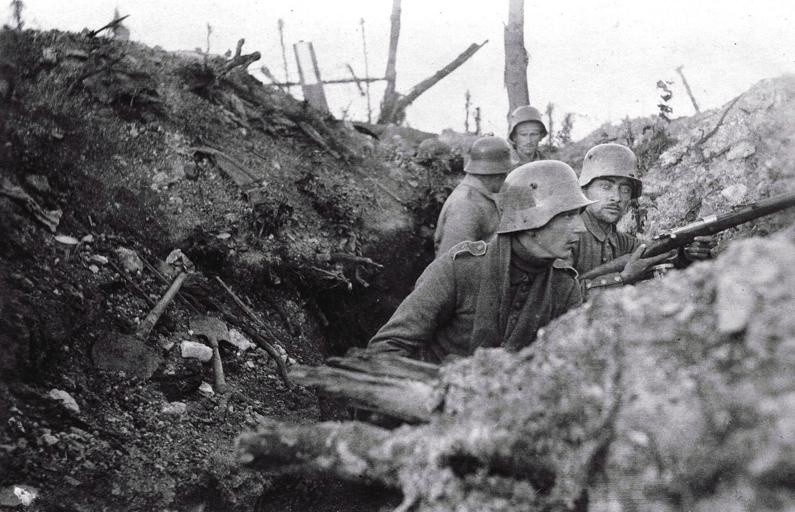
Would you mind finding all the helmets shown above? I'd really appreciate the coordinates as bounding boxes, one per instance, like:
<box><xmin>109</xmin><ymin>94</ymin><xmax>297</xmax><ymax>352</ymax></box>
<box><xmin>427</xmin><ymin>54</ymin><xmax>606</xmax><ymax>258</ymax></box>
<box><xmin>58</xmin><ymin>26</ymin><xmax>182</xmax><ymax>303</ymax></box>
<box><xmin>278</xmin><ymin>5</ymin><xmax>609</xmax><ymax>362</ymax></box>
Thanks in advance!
<box><xmin>507</xmin><ymin>107</ymin><xmax>548</xmax><ymax>140</ymax></box>
<box><xmin>497</xmin><ymin>159</ymin><xmax>599</xmax><ymax>233</ymax></box>
<box><xmin>416</xmin><ymin>138</ymin><xmax>451</xmax><ymax>164</ymax></box>
<box><xmin>578</xmin><ymin>143</ymin><xmax>643</xmax><ymax>198</ymax></box>
<box><xmin>464</xmin><ymin>136</ymin><xmax>513</xmax><ymax>176</ymax></box>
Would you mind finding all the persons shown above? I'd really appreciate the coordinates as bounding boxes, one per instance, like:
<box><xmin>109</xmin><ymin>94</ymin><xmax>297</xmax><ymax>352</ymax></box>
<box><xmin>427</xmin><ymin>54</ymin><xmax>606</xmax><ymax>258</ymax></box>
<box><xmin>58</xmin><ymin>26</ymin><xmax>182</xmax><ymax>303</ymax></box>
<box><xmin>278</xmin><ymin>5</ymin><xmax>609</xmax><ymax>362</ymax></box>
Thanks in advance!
<box><xmin>433</xmin><ymin>136</ymin><xmax>519</xmax><ymax>259</ymax></box>
<box><xmin>364</xmin><ymin>158</ymin><xmax>601</xmax><ymax>369</ymax></box>
<box><xmin>507</xmin><ymin>104</ymin><xmax>552</xmax><ymax>171</ymax></box>
<box><xmin>564</xmin><ymin>143</ymin><xmax>719</xmax><ymax>301</ymax></box>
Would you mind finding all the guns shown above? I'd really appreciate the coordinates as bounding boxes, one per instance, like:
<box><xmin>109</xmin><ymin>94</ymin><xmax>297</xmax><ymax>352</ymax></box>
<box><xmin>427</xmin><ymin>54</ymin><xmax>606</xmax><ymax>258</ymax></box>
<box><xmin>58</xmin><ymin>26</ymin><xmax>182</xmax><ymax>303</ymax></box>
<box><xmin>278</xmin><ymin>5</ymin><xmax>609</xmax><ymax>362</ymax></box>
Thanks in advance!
<box><xmin>577</xmin><ymin>191</ymin><xmax>795</xmax><ymax>280</ymax></box>
